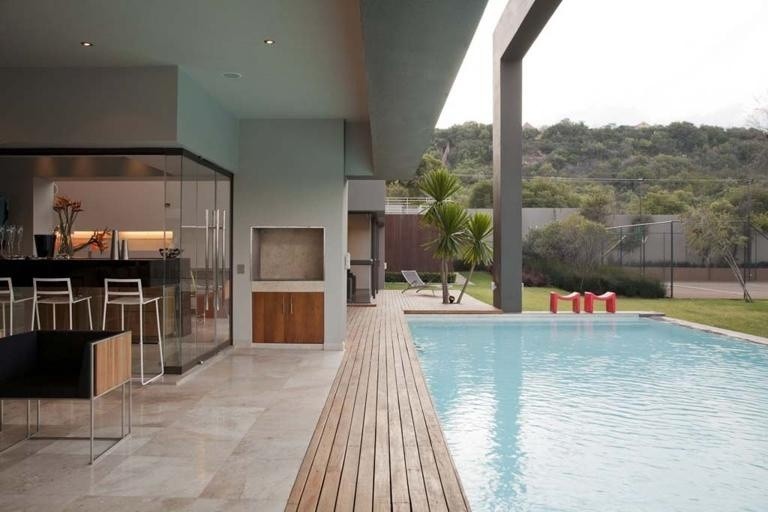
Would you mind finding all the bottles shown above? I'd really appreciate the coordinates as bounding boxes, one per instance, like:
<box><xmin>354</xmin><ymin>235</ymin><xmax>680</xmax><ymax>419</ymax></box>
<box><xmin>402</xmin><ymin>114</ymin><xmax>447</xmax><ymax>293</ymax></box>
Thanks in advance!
<box><xmin>110</xmin><ymin>230</ymin><xmax>120</xmax><ymax>260</ymax></box>
<box><xmin>120</xmin><ymin>239</ymin><xmax>128</xmax><ymax>260</ymax></box>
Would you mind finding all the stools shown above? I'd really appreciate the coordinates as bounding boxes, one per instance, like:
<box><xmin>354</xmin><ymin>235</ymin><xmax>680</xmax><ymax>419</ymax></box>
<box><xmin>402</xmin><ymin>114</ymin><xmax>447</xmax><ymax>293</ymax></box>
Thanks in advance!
<box><xmin>551</xmin><ymin>291</ymin><xmax>617</xmax><ymax>315</ymax></box>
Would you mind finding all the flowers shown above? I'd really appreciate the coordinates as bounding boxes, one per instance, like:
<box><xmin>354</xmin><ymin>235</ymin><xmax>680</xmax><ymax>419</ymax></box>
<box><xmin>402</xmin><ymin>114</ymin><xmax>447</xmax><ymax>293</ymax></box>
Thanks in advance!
<box><xmin>52</xmin><ymin>196</ymin><xmax>112</xmax><ymax>256</ymax></box>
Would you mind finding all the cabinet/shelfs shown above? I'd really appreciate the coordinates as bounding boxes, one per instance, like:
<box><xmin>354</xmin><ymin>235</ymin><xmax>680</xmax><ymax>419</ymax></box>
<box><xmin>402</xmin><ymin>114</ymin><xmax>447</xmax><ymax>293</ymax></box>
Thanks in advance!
<box><xmin>251</xmin><ymin>292</ymin><xmax>323</xmax><ymax>345</ymax></box>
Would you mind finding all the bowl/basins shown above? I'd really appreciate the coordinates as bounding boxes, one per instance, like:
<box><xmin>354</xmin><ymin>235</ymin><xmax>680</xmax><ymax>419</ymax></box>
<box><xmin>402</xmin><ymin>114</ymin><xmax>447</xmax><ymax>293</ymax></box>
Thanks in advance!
<box><xmin>158</xmin><ymin>248</ymin><xmax>184</xmax><ymax>258</ymax></box>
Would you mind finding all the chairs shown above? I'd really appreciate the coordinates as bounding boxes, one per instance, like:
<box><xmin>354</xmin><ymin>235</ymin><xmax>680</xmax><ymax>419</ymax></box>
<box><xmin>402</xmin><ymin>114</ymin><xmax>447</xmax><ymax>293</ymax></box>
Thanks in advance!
<box><xmin>1</xmin><ymin>329</ymin><xmax>131</xmax><ymax>470</ymax></box>
<box><xmin>401</xmin><ymin>270</ymin><xmax>439</xmax><ymax>298</ymax></box>
<box><xmin>0</xmin><ymin>276</ymin><xmax>167</xmax><ymax>388</ymax></box>
